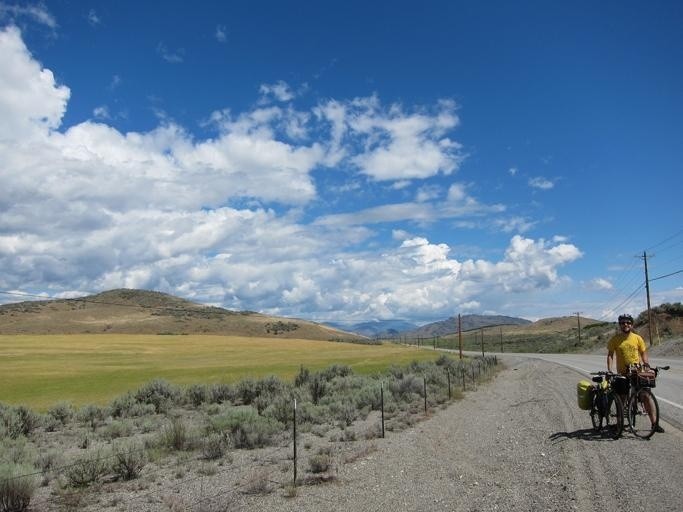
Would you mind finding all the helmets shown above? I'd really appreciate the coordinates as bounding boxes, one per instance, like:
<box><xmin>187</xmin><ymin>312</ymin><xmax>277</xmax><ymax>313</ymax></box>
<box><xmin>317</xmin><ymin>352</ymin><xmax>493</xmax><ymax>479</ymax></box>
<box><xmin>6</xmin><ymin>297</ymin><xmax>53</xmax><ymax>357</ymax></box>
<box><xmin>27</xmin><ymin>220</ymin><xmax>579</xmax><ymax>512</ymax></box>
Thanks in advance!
<box><xmin>618</xmin><ymin>314</ymin><xmax>634</xmax><ymax>323</ymax></box>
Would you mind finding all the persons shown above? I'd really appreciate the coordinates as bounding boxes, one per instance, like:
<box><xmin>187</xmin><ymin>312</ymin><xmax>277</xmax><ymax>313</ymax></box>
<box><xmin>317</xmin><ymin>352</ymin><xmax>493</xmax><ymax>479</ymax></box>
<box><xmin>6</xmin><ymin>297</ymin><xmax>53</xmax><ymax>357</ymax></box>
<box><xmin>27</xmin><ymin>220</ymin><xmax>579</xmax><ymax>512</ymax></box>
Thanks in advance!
<box><xmin>606</xmin><ymin>314</ymin><xmax>665</xmax><ymax>433</ymax></box>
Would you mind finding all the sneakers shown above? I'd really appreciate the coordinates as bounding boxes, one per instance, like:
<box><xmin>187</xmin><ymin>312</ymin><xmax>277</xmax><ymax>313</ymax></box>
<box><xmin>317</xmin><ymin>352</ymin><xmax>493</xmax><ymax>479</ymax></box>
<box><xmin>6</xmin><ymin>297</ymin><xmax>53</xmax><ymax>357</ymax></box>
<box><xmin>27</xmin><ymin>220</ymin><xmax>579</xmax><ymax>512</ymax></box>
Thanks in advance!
<box><xmin>651</xmin><ymin>424</ymin><xmax>664</xmax><ymax>433</ymax></box>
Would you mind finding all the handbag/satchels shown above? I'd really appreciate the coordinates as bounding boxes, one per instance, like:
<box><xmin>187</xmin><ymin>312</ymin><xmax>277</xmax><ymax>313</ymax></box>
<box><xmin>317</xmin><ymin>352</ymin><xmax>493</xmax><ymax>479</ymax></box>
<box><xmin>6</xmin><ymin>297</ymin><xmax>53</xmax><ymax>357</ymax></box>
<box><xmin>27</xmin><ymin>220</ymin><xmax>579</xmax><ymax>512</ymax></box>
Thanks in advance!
<box><xmin>577</xmin><ymin>380</ymin><xmax>594</xmax><ymax>410</ymax></box>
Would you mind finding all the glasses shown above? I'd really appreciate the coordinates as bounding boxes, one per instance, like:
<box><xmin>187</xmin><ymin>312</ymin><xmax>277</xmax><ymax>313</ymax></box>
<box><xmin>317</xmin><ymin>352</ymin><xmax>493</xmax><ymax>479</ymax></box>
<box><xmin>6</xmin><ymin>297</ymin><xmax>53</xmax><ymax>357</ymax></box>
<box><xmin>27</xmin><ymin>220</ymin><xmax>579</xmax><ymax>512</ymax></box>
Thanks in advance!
<box><xmin>621</xmin><ymin>321</ymin><xmax>632</xmax><ymax>325</ymax></box>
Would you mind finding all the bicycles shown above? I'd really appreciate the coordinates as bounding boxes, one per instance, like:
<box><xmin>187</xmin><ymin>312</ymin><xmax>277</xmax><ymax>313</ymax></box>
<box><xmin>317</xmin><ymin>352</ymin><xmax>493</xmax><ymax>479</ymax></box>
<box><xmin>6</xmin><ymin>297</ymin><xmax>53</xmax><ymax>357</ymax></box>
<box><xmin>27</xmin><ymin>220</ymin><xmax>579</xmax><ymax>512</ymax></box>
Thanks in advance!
<box><xmin>588</xmin><ymin>372</ymin><xmax>625</xmax><ymax>440</ymax></box>
<box><xmin>621</xmin><ymin>362</ymin><xmax>670</xmax><ymax>439</ymax></box>
